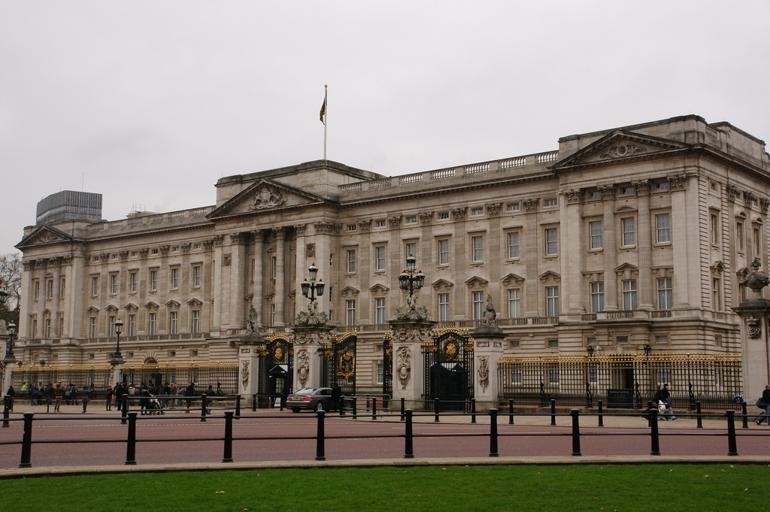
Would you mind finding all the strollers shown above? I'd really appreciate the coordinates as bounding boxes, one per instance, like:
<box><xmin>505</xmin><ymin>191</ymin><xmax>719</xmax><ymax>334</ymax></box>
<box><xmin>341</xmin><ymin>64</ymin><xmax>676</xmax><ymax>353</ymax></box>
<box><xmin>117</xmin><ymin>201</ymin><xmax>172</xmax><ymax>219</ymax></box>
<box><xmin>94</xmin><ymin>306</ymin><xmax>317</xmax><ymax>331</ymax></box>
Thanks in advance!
<box><xmin>148</xmin><ymin>393</ymin><xmax>164</xmax><ymax>416</ymax></box>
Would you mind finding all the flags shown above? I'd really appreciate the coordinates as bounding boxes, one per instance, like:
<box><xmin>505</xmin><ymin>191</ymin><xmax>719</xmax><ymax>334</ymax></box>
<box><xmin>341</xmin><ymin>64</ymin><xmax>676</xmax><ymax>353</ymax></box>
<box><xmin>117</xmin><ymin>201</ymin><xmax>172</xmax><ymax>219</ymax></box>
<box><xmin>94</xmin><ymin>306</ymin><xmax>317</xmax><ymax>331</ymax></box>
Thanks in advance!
<box><xmin>318</xmin><ymin>85</ymin><xmax>329</xmax><ymax>127</ymax></box>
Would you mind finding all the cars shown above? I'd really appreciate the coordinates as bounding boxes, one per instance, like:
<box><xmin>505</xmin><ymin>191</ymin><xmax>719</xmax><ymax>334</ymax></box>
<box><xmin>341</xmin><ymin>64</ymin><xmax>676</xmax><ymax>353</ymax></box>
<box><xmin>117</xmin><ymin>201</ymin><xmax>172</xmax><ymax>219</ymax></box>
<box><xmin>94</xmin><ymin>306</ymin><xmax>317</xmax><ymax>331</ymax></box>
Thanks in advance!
<box><xmin>285</xmin><ymin>387</ymin><xmax>353</xmax><ymax>412</ymax></box>
<box><xmin>65</xmin><ymin>385</ymin><xmax>92</xmax><ymax>399</ymax></box>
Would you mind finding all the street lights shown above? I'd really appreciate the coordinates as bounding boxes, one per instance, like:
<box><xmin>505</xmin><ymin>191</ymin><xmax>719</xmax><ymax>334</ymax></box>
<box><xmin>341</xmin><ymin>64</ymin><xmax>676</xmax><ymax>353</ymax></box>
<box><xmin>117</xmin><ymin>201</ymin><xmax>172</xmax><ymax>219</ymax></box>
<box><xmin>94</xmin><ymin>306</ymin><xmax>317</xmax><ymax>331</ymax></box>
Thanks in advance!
<box><xmin>300</xmin><ymin>262</ymin><xmax>324</xmax><ymax>315</ymax></box>
<box><xmin>113</xmin><ymin>317</ymin><xmax>124</xmax><ymax>351</ymax></box>
<box><xmin>587</xmin><ymin>343</ymin><xmax>594</xmax><ymax>357</ymax></box>
<box><xmin>5</xmin><ymin>320</ymin><xmax>16</xmax><ymax>354</ymax></box>
<box><xmin>399</xmin><ymin>252</ymin><xmax>426</xmax><ymax>309</ymax></box>
<box><xmin>643</xmin><ymin>342</ymin><xmax>651</xmax><ymax>355</ymax></box>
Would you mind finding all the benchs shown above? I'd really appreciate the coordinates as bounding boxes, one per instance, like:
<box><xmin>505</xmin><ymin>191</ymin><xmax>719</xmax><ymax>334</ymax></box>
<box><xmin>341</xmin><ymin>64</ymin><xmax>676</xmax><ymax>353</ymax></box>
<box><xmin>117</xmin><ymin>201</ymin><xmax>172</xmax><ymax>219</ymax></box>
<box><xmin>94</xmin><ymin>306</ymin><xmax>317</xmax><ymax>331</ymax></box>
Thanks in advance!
<box><xmin>11</xmin><ymin>398</ymin><xmax>107</xmax><ymax>406</ymax></box>
<box><xmin>129</xmin><ymin>399</ymin><xmax>235</xmax><ymax>408</ymax></box>
<box><xmin>500</xmin><ymin>403</ymin><xmax>731</xmax><ymax>419</ymax></box>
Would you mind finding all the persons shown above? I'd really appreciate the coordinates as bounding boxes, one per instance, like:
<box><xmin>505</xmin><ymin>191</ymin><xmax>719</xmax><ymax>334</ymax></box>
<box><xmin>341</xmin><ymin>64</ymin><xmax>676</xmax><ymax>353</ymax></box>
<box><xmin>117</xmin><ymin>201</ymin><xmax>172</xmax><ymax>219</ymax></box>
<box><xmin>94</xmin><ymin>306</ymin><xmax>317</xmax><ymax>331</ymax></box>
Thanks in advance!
<box><xmin>657</xmin><ymin>383</ymin><xmax>678</xmax><ymax>421</ymax></box>
<box><xmin>330</xmin><ymin>382</ymin><xmax>342</xmax><ymax>413</ymax></box>
<box><xmin>755</xmin><ymin>384</ymin><xmax>769</xmax><ymax>425</ymax></box>
<box><xmin>4</xmin><ymin>376</ymin><xmax>226</xmax><ymax>417</ymax></box>
